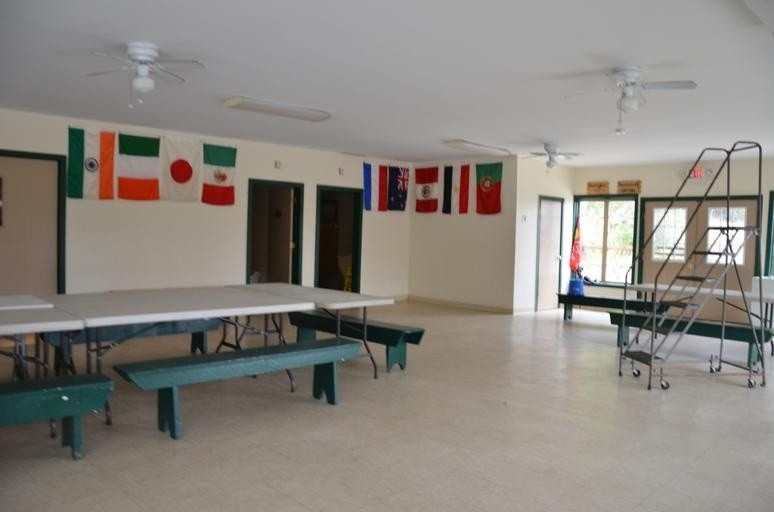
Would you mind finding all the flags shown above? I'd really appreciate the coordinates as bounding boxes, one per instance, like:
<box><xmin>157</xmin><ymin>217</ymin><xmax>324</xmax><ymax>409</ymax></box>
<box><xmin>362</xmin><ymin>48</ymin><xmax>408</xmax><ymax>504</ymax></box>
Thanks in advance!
<box><xmin>202</xmin><ymin>144</ymin><xmax>237</xmax><ymax>206</ymax></box>
<box><xmin>415</xmin><ymin>167</ymin><xmax>438</xmax><ymax>211</ymax></box>
<box><xmin>476</xmin><ymin>162</ymin><xmax>503</xmax><ymax>215</ymax></box>
<box><xmin>570</xmin><ymin>215</ymin><xmax>585</xmax><ymax>272</ymax></box>
<box><xmin>363</xmin><ymin>163</ymin><xmax>389</xmax><ymax>211</ymax></box>
<box><xmin>388</xmin><ymin>166</ymin><xmax>410</xmax><ymax>210</ymax></box>
<box><xmin>160</xmin><ymin>135</ymin><xmax>204</xmax><ymax>202</ymax></box>
<box><xmin>442</xmin><ymin>164</ymin><xmax>469</xmax><ymax>213</ymax></box>
<box><xmin>67</xmin><ymin>127</ymin><xmax>114</xmax><ymax>201</ymax></box>
<box><xmin>116</xmin><ymin>132</ymin><xmax>163</xmax><ymax>201</ymax></box>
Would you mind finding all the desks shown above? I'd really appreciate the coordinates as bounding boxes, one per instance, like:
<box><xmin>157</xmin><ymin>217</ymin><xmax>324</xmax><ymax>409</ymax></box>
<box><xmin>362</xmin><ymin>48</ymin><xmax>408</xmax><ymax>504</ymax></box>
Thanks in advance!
<box><xmin>0</xmin><ymin>284</ymin><xmax>315</xmax><ymax>442</ymax></box>
<box><xmin>227</xmin><ymin>281</ymin><xmax>394</xmax><ymax>380</ymax></box>
<box><xmin>630</xmin><ymin>284</ymin><xmax>773</xmax><ymax>363</ymax></box>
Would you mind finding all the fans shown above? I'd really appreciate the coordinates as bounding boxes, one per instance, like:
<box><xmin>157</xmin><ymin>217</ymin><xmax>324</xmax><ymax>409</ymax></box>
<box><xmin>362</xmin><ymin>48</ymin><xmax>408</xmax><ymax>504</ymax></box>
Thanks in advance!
<box><xmin>78</xmin><ymin>42</ymin><xmax>200</xmax><ymax>110</ymax></box>
<box><xmin>531</xmin><ymin>142</ymin><xmax>579</xmax><ymax>169</ymax></box>
<box><xmin>609</xmin><ymin>66</ymin><xmax>695</xmax><ymax>136</ymax></box>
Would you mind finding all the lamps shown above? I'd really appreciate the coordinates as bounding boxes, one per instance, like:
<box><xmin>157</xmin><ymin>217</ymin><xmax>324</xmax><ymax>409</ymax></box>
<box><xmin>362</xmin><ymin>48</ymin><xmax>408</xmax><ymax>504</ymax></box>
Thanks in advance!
<box><xmin>750</xmin><ymin>276</ymin><xmax>774</xmax><ymax>326</ymax></box>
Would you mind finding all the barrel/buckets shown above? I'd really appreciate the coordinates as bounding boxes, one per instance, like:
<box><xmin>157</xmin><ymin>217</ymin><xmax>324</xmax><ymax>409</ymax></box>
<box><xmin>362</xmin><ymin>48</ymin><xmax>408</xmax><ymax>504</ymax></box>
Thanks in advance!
<box><xmin>567</xmin><ymin>279</ymin><xmax>584</xmax><ymax>296</ymax></box>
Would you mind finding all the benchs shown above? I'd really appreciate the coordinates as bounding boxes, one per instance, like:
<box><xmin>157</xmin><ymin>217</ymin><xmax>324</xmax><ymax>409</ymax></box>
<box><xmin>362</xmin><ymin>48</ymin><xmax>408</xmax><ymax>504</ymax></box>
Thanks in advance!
<box><xmin>40</xmin><ymin>317</ymin><xmax>224</xmax><ymax>366</ymax></box>
<box><xmin>2</xmin><ymin>367</ymin><xmax>118</xmax><ymax>460</ymax></box>
<box><xmin>558</xmin><ymin>294</ymin><xmax>773</xmax><ymax>362</ymax></box>
<box><xmin>288</xmin><ymin>311</ymin><xmax>424</xmax><ymax>374</ymax></box>
<box><xmin>111</xmin><ymin>337</ymin><xmax>365</xmax><ymax>440</ymax></box>
